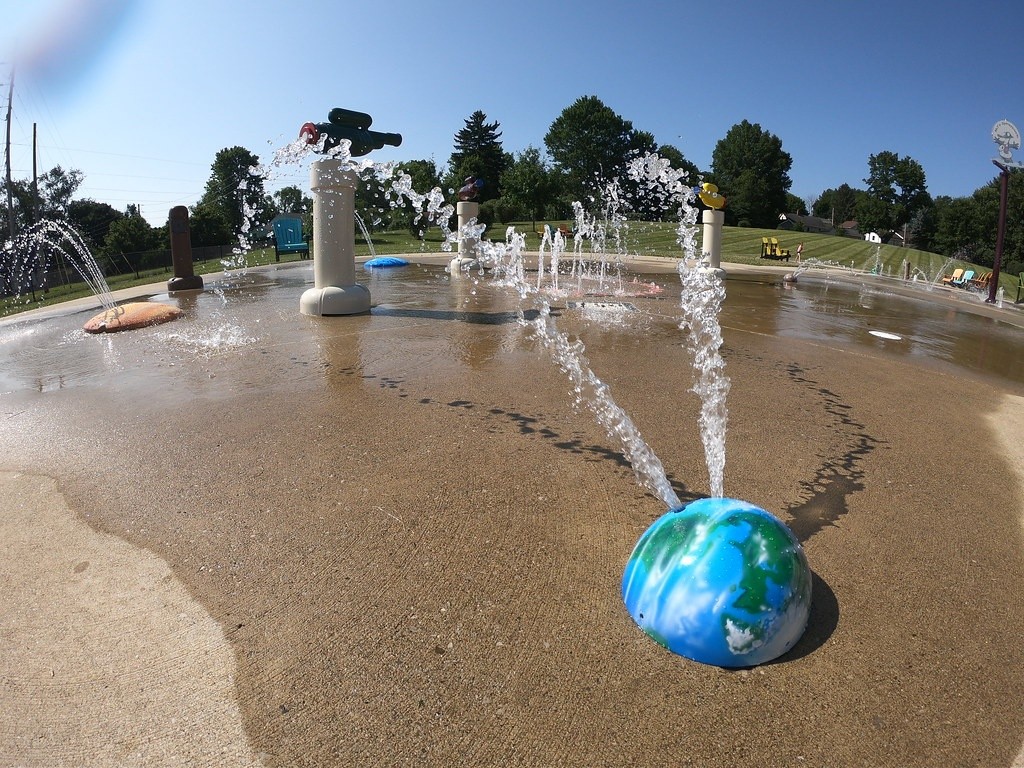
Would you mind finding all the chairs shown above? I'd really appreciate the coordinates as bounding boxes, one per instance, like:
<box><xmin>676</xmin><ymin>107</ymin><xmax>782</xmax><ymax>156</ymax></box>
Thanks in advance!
<box><xmin>760</xmin><ymin>236</ymin><xmax>791</xmax><ymax>262</ymax></box>
<box><xmin>536</xmin><ymin>223</ymin><xmax>575</xmax><ymax>239</ymax></box>
<box><xmin>271</xmin><ymin>215</ymin><xmax>311</xmax><ymax>261</ymax></box>
<box><xmin>1015</xmin><ymin>272</ymin><xmax>1024</xmax><ymax>303</ymax></box>
<box><xmin>943</xmin><ymin>269</ymin><xmax>992</xmax><ymax>292</ymax></box>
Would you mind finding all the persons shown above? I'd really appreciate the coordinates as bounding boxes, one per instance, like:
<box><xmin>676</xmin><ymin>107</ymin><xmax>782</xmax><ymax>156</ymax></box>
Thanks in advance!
<box><xmin>571</xmin><ymin>223</ymin><xmax>578</xmax><ymax>237</ymax></box>
<box><xmin>795</xmin><ymin>242</ymin><xmax>803</xmax><ymax>263</ymax></box>
